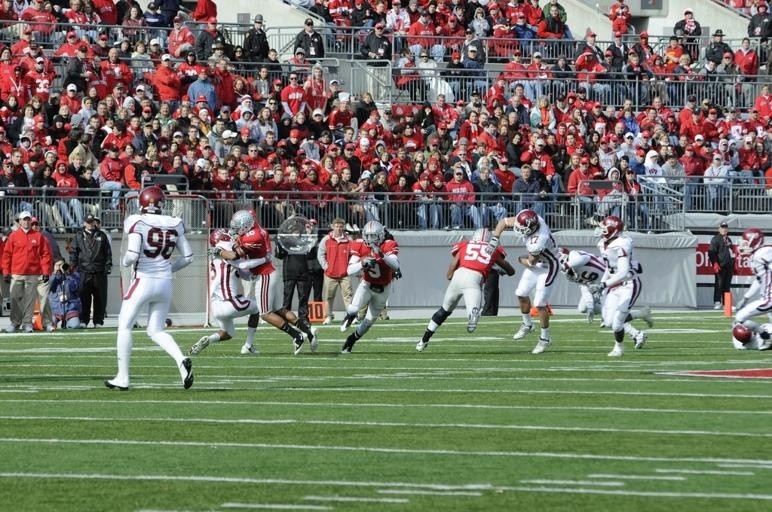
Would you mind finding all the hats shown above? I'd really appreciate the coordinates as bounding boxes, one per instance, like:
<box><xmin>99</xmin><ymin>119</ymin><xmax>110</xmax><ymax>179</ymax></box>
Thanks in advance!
<box><xmin>15</xmin><ymin>210</ymin><xmax>39</xmax><ymax>224</ymax></box>
<box><xmin>0</xmin><ymin>0</ymin><xmax>758</xmax><ymax>174</ymax></box>
<box><xmin>720</xmin><ymin>223</ymin><xmax>728</xmax><ymax>227</ymax></box>
<box><xmin>84</xmin><ymin>214</ymin><xmax>100</xmax><ymax>222</ymax></box>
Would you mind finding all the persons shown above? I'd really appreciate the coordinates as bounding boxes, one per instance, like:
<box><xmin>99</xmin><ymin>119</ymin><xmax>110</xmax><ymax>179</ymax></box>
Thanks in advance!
<box><xmin>186</xmin><ymin>226</ymin><xmax>274</xmax><ymax>358</ymax></box>
<box><xmin>414</xmin><ymin>226</ymin><xmax>515</xmax><ymax>351</ymax></box>
<box><xmin>484</xmin><ymin>207</ymin><xmax>561</xmax><ymax>355</ymax></box>
<box><xmin>726</xmin><ymin>321</ymin><xmax>771</xmax><ymax>350</ymax></box>
<box><xmin>209</xmin><ymin>207</ymin><xmax>318</xmax><ymax>355</ymax></box>
<box><xmin>597</xmin><ymin>217</ymin><xmax>648</xmax><ymax>358</ymax></box>
<box><xmin>103</xmin><ymin>186</ymin><xmax>196</xmax><ymax>392</ymax></box>
<box><xmin>0</xmin><ymin>1</ymin><xmax>771</xmax><ymax>334</ymax></box>
<box><xmin>338</xmin><ymin>219</ymin><xmax>402</xmax><ymax>356</ymax></box>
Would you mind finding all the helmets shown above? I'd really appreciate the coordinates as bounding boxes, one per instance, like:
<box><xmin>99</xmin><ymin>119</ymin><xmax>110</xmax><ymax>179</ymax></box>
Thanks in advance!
<box><xmin>473</xmin><ymin>228</ymin><xmax>491</xmax><ymax>241</ymax></box>
<box><xmin>593</xmin><ymin>216</ymin><xmax>623</xmax><ymax>242</ymax></box>
<box><xmin>733</xmin><ymin>323</ymin><xmax>752</xmax><ymax>343</ymax></box>
<box><xmin>513</xmin><ymin>209</ymin><xmax>538</xmax><ymax>237</ymax></box>
<box><xmin>362</xmin><ymin>221</ymin><xmax>382</xmax><ymax>247</ymax></box>
<box><xmin>741</xmin><ymin>228</ymin><xmax>763</xmax><ymax>253</ymax></box>
<box><xmin>209</xmin><ymin>210</ymin><xmax>256</xmax><ymax>247</ymax></box>
<box><xmin>138</xmin><ymin>186</ymin><xmax>165</xmax><ymax>214</ymax></box>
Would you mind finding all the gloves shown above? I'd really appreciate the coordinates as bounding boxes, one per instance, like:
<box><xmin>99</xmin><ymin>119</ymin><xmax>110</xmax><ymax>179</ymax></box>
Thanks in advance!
<box><xmin>485</xmin><ymin>237</ymin><xmax>499</xmax><ymax>255</ymax></box>
<box><xmin>713</xmin><ymin>263</ymin><xmax>721</xmax><ymax>273</ymax></box>
<box><xmin>589</xmin><ymin>283</ymin><xmax>604</xmax><ymax>298</ymax></box>
<box><xmin>362</xmin><ymin>244</ymin><xmax>382</xmax><ymax>271</ymax></box>
<box><xmin>736</xmin><ymin>297</ymin><xmax>746</xmax><ymax>310</ymax></box>
<box><xmin>586</xmin><ymin>309</ymin><xmax>595</xmax><ymax>324</ymax></box>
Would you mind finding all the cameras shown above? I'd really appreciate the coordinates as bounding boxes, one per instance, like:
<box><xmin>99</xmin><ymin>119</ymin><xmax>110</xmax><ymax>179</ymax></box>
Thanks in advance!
<box><xmin>59</xmin><ymin>263</ymin><xmax>69</xmax><ymax>274</ymax></box>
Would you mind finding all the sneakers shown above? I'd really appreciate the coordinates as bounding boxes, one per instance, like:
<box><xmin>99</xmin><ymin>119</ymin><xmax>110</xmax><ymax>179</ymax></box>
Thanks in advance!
<box><xmin>514</xmin><ymin>322</ymin><xmax>534</xmax><ymax>340</ymax></box>
<box><xmin>179</xmin><ymin>357</ymin><xmax>194</xmax><ymax>389</ymax></box>
<box><xmin>600</xmin><ymin>305</ymin><xmax>653</xmax><ymax>356</ymax></box>
<box><xmin>189</xmin><ymin>336</ymin><xmax>209</xmax><ymax>355</ymax></box>
<box><xmin>6</xmin><ymin>322</ymin><xmax>103</xmax><ymax>333</ymax></box>
<box><xmin>416</xmin><ymin>338</ymin><xmax>429</xmax><ymax>351</ymax></box>
<box><xmin>468</xmin><ymin>307</ymin><xmax>479</xmax><ymax>332</ymax></box>
<box><xmin>105</xmin><ymin>379</ymin><xmax>128</xmax><ymax>391</ymax></box>
<box><xmin>714</xmin><ymin>301</ymin><xmax>735</xmax><ymax>310</ymax></box>
<box><xmin>531</xmin><ymin>336</ymin><xmax>550</xmax><ymax>354</ymax></box>
<box><xmin>241</xmin><ymin>316</ymin><xmax>361</xmax><ymax>354</ymax></box>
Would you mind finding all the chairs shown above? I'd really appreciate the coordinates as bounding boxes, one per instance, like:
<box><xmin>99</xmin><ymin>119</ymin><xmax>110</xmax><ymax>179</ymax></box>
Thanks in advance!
<box><xmin>0</xmin><ymin>0</ymin><xmax>772</xmax><ymax>238</ymax></box>
